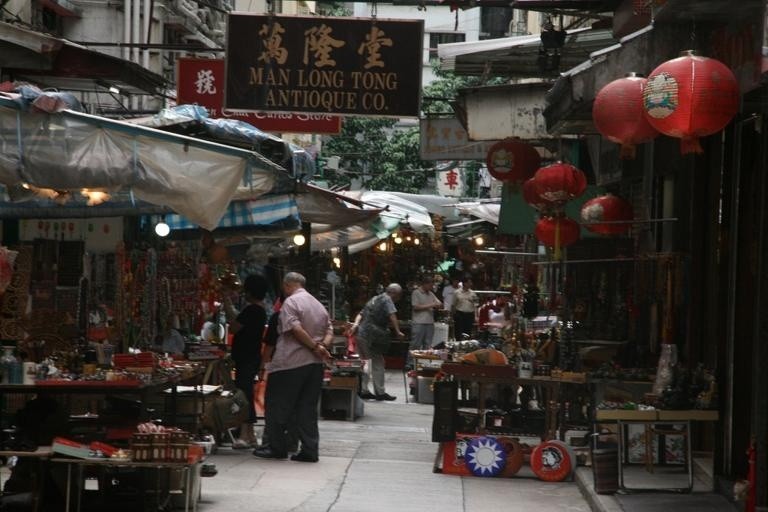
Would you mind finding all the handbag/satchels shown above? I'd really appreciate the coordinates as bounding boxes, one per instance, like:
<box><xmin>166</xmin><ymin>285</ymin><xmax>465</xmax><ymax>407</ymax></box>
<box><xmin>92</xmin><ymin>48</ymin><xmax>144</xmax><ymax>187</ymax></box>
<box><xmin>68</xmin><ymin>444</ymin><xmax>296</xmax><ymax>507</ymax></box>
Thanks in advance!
<box><xmin>367</xmin><ymin>324</ymin><xmax>392</xmax><ymax>353</ymax></box>
<box><xmin>202</xmin><ymin>389</ymin><xmax>251</xmax><ymax>434</ymax></box>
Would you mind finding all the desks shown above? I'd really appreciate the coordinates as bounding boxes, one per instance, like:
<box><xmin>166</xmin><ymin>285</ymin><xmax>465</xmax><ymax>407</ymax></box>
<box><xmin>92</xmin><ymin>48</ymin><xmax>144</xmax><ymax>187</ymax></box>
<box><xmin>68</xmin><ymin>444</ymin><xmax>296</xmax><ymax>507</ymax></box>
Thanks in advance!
<box><xmin>408</xmin><ymin>348</ymin><xmax>562</xmax><ymax>480</ymax></box>
<box><xmin>0</xmin><ymin>362</ymin><xmax>223</xmax><ymax>512</ymax></box>
<box><xmin>591</xmin><ymin>407</ymin><xmax>721</xmax><ymax>497</ymax></box>
<box><xmin>317</xmin><ymin>375</ymin><xmax>359</xmax><ymax>422</ymax></box>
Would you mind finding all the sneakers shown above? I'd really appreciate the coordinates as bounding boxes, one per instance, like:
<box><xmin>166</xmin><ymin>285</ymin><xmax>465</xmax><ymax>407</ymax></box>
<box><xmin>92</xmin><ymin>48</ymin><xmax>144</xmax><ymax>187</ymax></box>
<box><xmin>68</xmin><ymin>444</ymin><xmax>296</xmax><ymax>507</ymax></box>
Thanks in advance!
<box><xmin>360</xmin><ymin>391</ymin><xmax>376</xmax><ymax>400</ymax></box>
<box><xmin>233</xmin><ymin>435</ymin><xmax>256</xmax><ymax>449</ymax></box>
<box><xmin>290</xmin><ymin>452</ymin><xmax>319</xmax><ymax>462</ymax></box>
<box><xmin>252</xmin><ymin>444</ymin><xmax>289</xmax><ymax>459</ymax></box>
<box><xmin>376</xmin><ymin>393</ymin><xmax>397</xmax><ymax>401</ymax></box>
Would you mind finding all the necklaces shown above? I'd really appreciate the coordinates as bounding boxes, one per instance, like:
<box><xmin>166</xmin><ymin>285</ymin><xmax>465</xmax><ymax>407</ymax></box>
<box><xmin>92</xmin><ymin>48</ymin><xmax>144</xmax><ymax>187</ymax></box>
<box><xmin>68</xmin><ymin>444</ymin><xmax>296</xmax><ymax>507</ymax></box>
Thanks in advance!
<box><xmin>74</xmin><ymin>242</ymin><xmax>174</xmax><ymax>348</ymax></box>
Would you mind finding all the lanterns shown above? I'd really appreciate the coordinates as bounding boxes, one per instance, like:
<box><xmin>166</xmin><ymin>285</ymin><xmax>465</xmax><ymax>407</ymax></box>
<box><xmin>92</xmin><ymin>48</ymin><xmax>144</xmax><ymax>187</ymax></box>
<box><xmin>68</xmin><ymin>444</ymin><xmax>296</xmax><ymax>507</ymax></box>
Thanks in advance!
<box><xmin>487</xmin><ymin>135</ymin><xmax>538</xmax><ymax>189</ymax></box>
<box><xmin>640</xmin><ymin>48</ymin><xmax>738</xmax><ymax>154</ymax></box>
<box><xmin>593</xmin><ymin>72</ymin><xmax>655</xmax><ymax>157</ymax></box>
<box><xmin>523</xmin><ymin>158</ymin><xmax>634</xmax><ymax>263</ymax></box>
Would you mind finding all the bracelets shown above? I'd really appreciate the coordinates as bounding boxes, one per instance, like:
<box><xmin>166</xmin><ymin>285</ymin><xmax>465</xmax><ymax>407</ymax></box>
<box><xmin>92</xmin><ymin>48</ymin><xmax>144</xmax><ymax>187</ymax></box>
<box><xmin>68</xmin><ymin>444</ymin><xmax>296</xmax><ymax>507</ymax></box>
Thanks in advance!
<box><xmin>258</xmin><ymin>368</ymin><xmax>267</xmax><ymax>372</ymax></box>
<box><xmin>320</xmin><ymin>342</ymin><xmax>327</xmax><ymax>350</ymax></box>
<box><xmin>312</xmin><ymin>342</ymin><xmax>319</xmax><ymax>352</ymax></box>
<box><xmin>174</xmin><ymin>249</ymin><xmax>203</xmax><ymax>316</ymax></box>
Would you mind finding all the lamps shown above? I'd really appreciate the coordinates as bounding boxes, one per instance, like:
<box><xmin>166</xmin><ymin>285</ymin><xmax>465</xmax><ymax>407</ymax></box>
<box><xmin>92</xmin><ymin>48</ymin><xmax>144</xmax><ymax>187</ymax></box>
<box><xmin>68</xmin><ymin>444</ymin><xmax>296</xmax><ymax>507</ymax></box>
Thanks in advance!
<box><xmin>21</xmin><ymin>182</ymin><xmax>112</xmax><ymax>208</ymax></box>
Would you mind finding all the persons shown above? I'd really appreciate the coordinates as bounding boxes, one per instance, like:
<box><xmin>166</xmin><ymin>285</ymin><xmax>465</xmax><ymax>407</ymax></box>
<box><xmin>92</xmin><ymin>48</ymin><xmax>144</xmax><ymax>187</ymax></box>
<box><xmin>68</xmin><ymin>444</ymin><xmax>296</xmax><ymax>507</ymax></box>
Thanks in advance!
<box><xmin>256</xmin><ymin>298</ymin><xmax>296</xmax><ymax>459</ymax></box>
<box><xmin>254</xmin><ymin>271</ymin><xmax>333</xmax><ymax>462</ymax></box>
<box><xmin>346</xmin><ymin>283</ymin><xmax>405</xmax><ymax>400</ymax></box>
<box><xmin>403</xmin><ymin>275</ymin><xmax>441</xmax><ymax>371</ymax></box>
<box><xmin>442</xmin><ymin>277</ymin><xmax>459</xmax><ymax>339</ymax></box>
<box><xmin>453</xmin><ymin>436</ymin><xmax>473</xmax><ymax>466</ymax></box>
<box><xmin>224</xmin><ymin>275</ymin><xmax>266</xmax><ymax>449</ymax></box>
<box><xmin>538</xmin><ymin>446</ymin><xmax>562</xmax><ymax>472</ymax></box>
<box><xmin>479</xmin><ymin>288</ymin><xmax>512</xmax><ymax>337</ymax></box>
<box><xmin>450</xmin><ymin>278</ymin><xmax>478</xmax><ymax>341</ymax></box>
